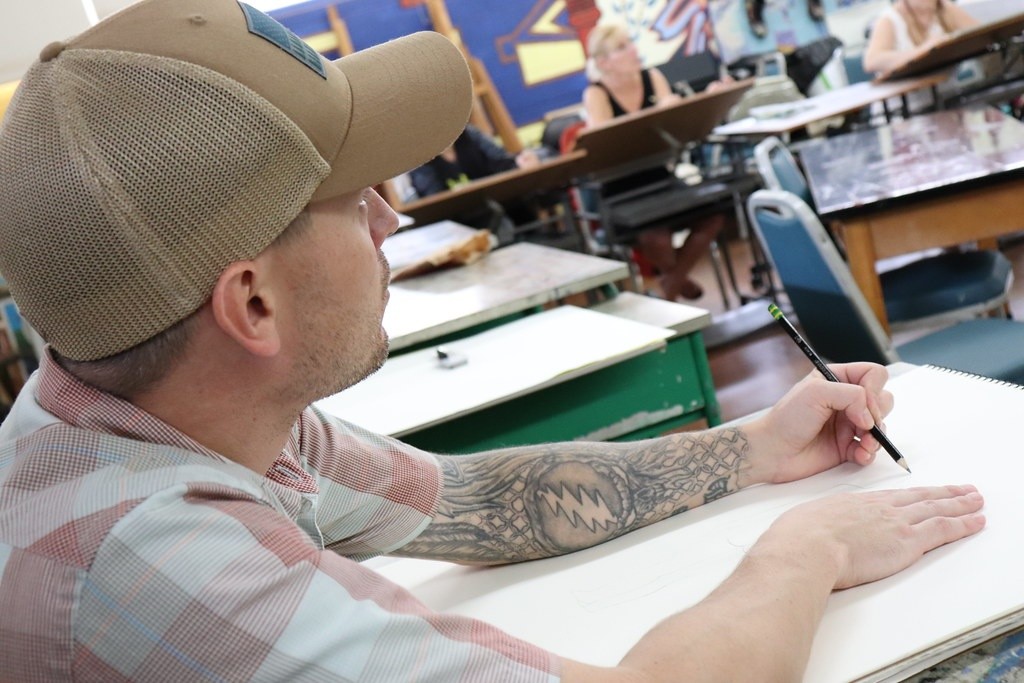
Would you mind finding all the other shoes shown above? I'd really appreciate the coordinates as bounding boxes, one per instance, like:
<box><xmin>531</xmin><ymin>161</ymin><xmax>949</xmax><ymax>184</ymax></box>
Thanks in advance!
<box><xmin>676</xmin><ymin>272</ymin><xmax>702</xmax><ymax>299</ymax></box>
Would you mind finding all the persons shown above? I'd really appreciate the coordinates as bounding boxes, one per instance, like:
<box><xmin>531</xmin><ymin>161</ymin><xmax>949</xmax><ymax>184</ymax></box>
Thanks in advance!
<box><xmin>863</xmin><ymin>0</ymin><xmax>985</xmax><ymax>128</ymax></box>
<box><xmin>582</xmin><ymin>22</ymin><xmax>727</xmax><ymax>302</ymax></box>
<box><xmin>410</xmin><ymin>125</ymin><xmax>541</xmax><ymax>244</ymax></box>
<box><xmin>0</xmin><ymin>0</ymin><xmax>986</xmax><ymax>683</ymax></box>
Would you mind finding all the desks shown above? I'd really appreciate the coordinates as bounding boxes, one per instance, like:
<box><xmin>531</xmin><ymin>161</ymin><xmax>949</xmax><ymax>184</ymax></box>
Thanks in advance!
<box><xmin>313</xmin><ymin>0</ymin><xmax>1024</xmax><ymax>683</ymax></box>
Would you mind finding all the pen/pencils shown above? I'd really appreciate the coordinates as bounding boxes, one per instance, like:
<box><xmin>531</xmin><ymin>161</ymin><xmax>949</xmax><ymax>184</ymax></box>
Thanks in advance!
<box><xmin>767</xmin><ymin>303</ymin><xmax>912</xmax><ymax>474</ymax></box>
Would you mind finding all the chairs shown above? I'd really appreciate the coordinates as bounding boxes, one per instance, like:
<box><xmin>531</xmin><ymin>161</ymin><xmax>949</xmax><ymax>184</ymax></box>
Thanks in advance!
<box><xmin>750</xmin><ymin>135</ymin><xmax>1024</xmax><ymax>332</ymax></box>
<box><xmin>563</xmin><ymin>121</ymin><xmax>742</xmax><ymax>314</ymax></box>
<box><xmin>746</xmin><ymin>189</ymin><xmax>1024</xmax><ymax>386</ymax></box>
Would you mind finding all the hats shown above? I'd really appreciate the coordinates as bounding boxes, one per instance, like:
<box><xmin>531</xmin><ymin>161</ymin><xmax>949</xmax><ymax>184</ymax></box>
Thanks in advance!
<box><xmin>0</xmin><ymin>0</ymin><xmax>469</xmax><ymax>362</ymax></box>
